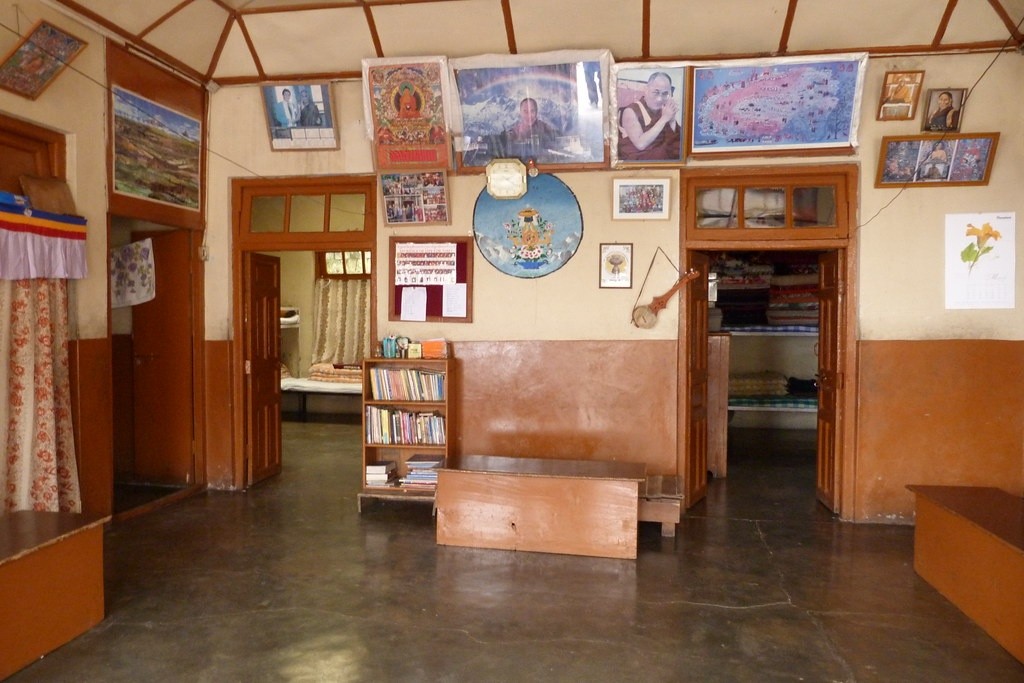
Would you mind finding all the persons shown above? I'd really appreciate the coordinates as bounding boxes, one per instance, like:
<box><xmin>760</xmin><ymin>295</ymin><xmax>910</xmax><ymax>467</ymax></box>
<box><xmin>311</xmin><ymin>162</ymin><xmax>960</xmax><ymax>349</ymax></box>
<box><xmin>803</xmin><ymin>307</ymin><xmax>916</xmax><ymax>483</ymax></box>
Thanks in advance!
<box><xmin>502</xmin><ymin>98</ymin><xmax>568</xmax><ymax>145</ymax></box>
<box><xmin>619</xmin><ymin>73</ymin><xmax>683</xmax><ymax>160</ymax></box>
<box><xmin>384</xmin><ymin>175</ymin><xmax>448</xmax><ymax>223</ymax></box>
<box><xmin>277</xmin><ymin>89</ymin><xmax>303</xmax><ymax>128</ymax></box>
<box><xmin>294</xmin><ymin>90</ymin><xmax>323</xmax><ymax>129</ymax></box>
<box><xmin>620</xmin><ymin>186</ymin><xmax>664</xmax><ymax>214</ymax></box>
<box><xmin>920</xmin><ymin>142</ymin><xmax>950</xmax><ymax>181</ymax></box>
<box><xmin>891</xmin><ymin>80</ymin><xmax>911</xmax><ymax>103</ymax></box>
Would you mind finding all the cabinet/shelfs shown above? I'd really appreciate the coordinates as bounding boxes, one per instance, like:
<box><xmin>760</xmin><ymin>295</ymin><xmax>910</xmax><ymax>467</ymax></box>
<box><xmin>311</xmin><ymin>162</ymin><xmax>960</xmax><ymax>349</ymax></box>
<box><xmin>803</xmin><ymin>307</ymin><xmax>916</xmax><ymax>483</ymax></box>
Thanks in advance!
<box><xmin>430</xmin><ymin>453</ymin><xmax>648</xmax><ymax>560</ymax></box>
<box><xmin>903</xmin><ymin>483</ymin><xmax>1024</xmax><ymax>662</ymax></box>
<box><xmin>707</xmin><ymin>330</ymin><xmax>733</xmax><ymax>478</ymax></box>
<box><xmin>0</xmin><ymin>509</ymin><xmax>114</xmax><ymax>681</ymax></box>
<box><xmin>357</xmin><ymin>357</ymin><xmax>457</xmax><ymax>517</ymax></box>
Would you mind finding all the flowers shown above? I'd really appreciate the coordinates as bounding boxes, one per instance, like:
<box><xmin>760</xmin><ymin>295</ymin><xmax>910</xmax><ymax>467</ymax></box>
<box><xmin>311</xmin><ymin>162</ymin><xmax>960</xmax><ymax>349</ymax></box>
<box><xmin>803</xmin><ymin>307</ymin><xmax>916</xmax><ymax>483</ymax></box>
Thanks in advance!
<box><xmin>961</xmin><ymin>223</ymin><xmax>1003</xmax><ymax>277</ymax></box>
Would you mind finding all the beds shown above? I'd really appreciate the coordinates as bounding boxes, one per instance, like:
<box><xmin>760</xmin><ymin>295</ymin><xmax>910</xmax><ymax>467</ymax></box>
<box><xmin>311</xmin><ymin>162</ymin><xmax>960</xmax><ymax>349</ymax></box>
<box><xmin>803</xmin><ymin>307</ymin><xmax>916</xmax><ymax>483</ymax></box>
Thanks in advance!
<box><xmin>280</xmin><ymin>377</ymin><xmax>363</xmax><ymax>422</ymax></box>
<box><xmin>728</xmin><ymin>392</ymin><xmax>818</xmax><ymax>412</ymax></box>
<box><xmin>720</xmin><ymin>323</ymin><xmax>819</xmax><ymax>337</ymax></box>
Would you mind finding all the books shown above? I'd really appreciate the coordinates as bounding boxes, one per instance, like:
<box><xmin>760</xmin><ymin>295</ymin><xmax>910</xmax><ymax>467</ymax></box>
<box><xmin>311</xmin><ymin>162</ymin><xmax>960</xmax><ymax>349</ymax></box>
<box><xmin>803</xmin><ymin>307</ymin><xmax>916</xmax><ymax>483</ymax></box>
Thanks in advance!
<box><xmin>365</xmin><ymin>366</ymin><xmax>447</xmax><ymax>489</ymax></box>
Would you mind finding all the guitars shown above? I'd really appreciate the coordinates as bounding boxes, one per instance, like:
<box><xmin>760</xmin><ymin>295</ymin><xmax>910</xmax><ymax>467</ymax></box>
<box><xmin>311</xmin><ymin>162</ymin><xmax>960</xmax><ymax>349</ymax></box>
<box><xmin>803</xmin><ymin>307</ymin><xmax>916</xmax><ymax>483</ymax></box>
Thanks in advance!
<box><xmin>632</xmin><ymin>267</ymin><xmax>700</xmax><ymax>329</ymax></box>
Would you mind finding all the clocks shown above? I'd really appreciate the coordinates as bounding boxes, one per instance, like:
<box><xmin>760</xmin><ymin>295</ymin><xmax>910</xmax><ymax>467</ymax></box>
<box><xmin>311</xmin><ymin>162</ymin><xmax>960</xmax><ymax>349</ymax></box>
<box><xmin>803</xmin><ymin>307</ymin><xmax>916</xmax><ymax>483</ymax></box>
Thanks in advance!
<box><xmin>485</xmin><ymin>157</ymin><xmax>527</xmax><ymax>200</ymax></box>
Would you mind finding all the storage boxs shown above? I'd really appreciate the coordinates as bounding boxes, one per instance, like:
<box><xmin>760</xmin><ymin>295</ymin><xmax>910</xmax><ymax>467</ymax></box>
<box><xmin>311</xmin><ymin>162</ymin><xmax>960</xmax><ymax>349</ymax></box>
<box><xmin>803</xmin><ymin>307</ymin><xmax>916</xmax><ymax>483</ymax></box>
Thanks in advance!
<box><xmin>707</xmin><ymin>307</ymin><xmax>723</xmax><ymax>332</ymax></box>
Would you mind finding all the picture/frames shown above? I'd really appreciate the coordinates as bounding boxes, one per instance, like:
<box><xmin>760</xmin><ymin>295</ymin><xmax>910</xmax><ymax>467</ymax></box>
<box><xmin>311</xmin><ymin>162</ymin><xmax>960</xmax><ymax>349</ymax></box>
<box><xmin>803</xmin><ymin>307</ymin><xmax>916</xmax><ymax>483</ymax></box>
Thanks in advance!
<box><xmin>361</xmin><ymin>55</ymin><xmax>453</xmax><ymax>172</ymax></box>
<box><xmin>610</xmin><ymin>61</ymin><xmax>690</xmax><ymax>171</ymax></box>
<box><xmin>388</xmin><ymin>235</ymin><xmax>474</xmax><ymax>324</ymax></box>
<box><xmin>876</xmin><ymin>70</ymin><xmax>926</xmax><ymax>122</ymax></box>
<box><xmin>874</xmin><ymin>132</ymin><xmax>1001</xmax><ymax>188</ymax></box>
<box><xmin>610</xmin><ymin>176</ymin><xmax>671</xmax><ymax>220</ymax></box>
<box><xmin>689</xmin><ymin>51</ymin><xmax>870</xmax><ymax>162</ymax></box>
<box><xmin>920</xmin><ymin>87</ymin><xmax>968</xmax><ymax>133</ymax></box>
<box><xmin>259</xmin><ymin>78</ymin><xmax>341</xmax><ymax>152</ymax></box>
<box><xmin>448</xmin><ymin>48</ymin><xmax>616</xmax><ymax>175</ymax></box>
<box><xmin>0</xmin><ymin>18</ymin><xmax>89</xmax><ymax>101</ymax></box>
<box><xmin>599</xmin><ymin>243</ymin><xmax>633</xmax><ymax>289</ymax></box>
